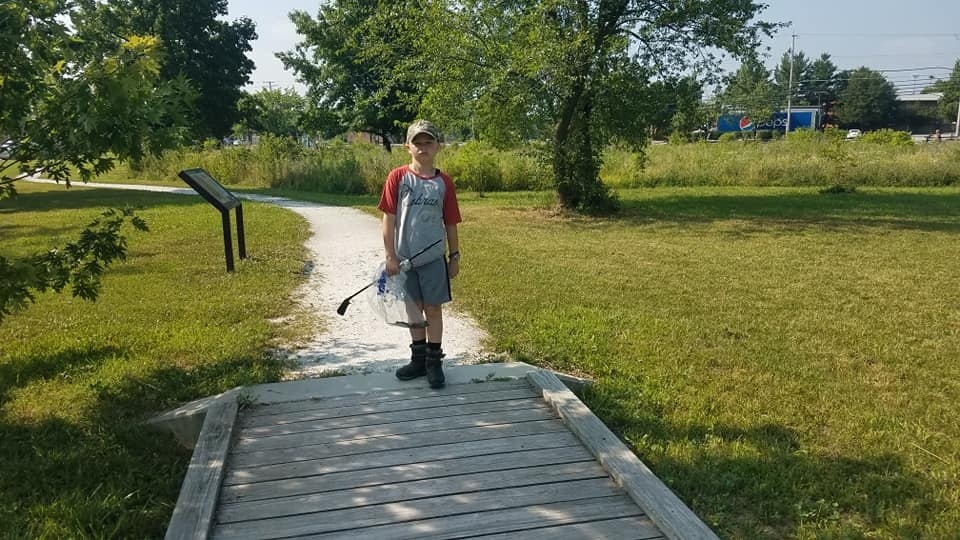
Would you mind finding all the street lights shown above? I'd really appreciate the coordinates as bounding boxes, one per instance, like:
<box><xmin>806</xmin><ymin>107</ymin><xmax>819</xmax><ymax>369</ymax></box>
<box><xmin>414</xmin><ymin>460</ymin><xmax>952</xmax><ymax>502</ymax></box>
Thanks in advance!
<box><xmin>784</xmin><ymin>34</ymin><xmax>799</xmax><ymax>136</ymax></box>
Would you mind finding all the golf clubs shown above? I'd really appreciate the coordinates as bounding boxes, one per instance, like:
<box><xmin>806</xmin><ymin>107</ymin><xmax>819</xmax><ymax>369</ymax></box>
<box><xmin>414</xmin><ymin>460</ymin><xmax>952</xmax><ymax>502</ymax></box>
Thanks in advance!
<box><xmin>336</xmin><ymin>238</ymin><xmax>443</xmax><ymax>316</ymax></box>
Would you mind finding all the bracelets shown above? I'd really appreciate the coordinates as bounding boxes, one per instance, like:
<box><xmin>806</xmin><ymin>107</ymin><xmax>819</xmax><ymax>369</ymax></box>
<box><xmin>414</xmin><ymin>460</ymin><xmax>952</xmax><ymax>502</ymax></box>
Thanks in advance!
<box><xmin>448</xmin><ymin>251</ymin><xmax>461</xmax><ymax>260</ymax></box>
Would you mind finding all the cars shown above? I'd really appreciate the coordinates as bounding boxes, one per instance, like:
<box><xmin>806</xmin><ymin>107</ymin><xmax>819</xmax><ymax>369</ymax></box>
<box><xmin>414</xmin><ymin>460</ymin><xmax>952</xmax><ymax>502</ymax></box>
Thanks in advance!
<box><xmin>846</xmin><ymin>128</ymin><xmax>862</xmax><ymax>139</ymax></box>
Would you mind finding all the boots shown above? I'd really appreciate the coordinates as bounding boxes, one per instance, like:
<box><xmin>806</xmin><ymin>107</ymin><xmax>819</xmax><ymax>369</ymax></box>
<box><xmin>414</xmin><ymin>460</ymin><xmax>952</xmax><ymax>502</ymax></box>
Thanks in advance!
<box><xmin>396</xmin><ymin>343</ymin><xmax>427</xmax><ymax>381</ymax></box>
<box><xmin>424</xmin><ymin>349</ymin><xmax>446</xmax><ymax>387</ymax></box>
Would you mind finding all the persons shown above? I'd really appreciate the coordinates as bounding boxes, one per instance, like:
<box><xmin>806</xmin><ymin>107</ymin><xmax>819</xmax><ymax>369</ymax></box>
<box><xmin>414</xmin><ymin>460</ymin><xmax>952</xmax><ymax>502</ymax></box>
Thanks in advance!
<box><xmin>377</xmin><ymin>121</ymin><xmax>462</xmax><ymax>388</ymax></box>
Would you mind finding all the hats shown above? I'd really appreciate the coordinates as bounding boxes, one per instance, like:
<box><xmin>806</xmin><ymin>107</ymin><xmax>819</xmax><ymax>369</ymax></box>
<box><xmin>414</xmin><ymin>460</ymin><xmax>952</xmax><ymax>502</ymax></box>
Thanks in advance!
<box><xmin>406</xmin><ymin>121</ymin><xmax>438</xmax><ymax>143</ymax></box>
